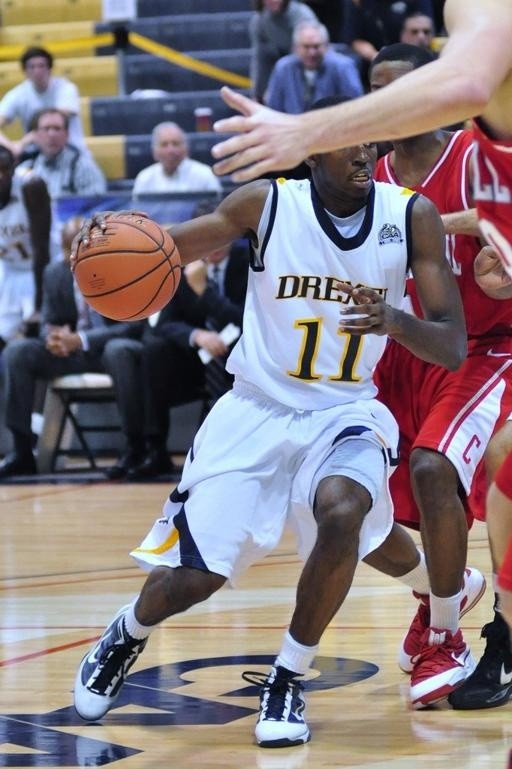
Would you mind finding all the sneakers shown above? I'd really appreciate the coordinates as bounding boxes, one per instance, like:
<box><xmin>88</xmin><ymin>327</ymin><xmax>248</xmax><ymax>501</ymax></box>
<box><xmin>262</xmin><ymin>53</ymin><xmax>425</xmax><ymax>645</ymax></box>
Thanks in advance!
<box><xmin>74</xmin><ymin>603</ymin><xmax>150</xmax><ymax>719</ymax></box>
<box><xmin>447</xmin><ymin>611</ymin><xmax>510</xmax><ymax>708</ymax></box>
<box><xmin>407</xmin><ymin>626</ymin><xmax>478</xmax><ymax>710</ymax></box>
<box><xmin>240</xmin><ymin>664</ymin><xmax>320</xmax><ymax>748</ymax></box>
<box><xmin>398</xmin><ymin>568</ymin><xmax>486</xmax><ymax>674</ymax></box>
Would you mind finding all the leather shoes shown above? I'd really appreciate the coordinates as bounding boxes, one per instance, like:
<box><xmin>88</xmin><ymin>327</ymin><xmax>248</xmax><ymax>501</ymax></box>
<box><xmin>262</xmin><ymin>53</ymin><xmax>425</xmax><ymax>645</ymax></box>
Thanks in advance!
<box><xmin>0</xmin><ymin>447</ymin><xmax>37</xmax><ymax>478</ymax></box>
<box><xmin>104</xmin><ymin>440</ymin><xmax>143</xmax><ymax>480</ymax></box>
<box><xmin>127</xmin><ymin>442</ymin><xmax>175</xmax><ymax>482</ymax></box>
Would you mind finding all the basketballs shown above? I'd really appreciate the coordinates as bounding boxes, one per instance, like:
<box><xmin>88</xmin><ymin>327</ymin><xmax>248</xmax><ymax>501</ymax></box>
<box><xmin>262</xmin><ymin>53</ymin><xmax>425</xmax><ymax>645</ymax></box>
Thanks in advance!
<box><xmin>76</xmin><ymin>213</ymin><xmax>181</xmax><ymax>322</ymax></box>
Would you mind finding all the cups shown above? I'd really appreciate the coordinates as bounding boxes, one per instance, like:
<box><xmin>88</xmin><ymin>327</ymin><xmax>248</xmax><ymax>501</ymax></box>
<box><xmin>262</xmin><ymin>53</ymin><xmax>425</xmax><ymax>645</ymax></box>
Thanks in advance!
<box><xmin>193</xmin><ymin>107</ymin><xmax>212</xmax><ymax>132</ymax></box>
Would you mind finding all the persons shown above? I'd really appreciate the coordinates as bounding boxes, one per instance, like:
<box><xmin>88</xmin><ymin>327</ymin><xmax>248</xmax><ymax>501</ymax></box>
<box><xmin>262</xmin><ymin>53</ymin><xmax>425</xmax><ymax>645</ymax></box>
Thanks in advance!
<box><xmin>7</xmin><ymin>107</ymin><xmax>99</xmax><ymax>203</ymax></box>
<box><xmin>0</xmin><ymin>214</ymin><xmax>147</xmax><ymax>480</ymax></box>
<box><xmin>0</xmin><ymin>45</ymin><xmax>83</xmax><ymax>150</ymax></box>
<box><xmin>128</xmin><ymin>120</ymin><xmax>224</xmax><ymax>213</ymax></box>
<box><xmin>126</xmin><ymin>213</ymin><xmax>246</xmax><ymax>471</ymax></box>
<box><xmin>70</xmin><ymin>101</ymin><xmax>467</xmax><ymax>748</ymax></box>
<box><xmin>211</xmin><ymin>0</ymin><xmax>512</xmax><ymax>709</ymax></box>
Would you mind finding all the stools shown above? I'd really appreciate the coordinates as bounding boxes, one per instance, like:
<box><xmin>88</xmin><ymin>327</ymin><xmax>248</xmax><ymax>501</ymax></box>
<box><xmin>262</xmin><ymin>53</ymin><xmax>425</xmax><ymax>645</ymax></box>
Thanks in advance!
<box><xmin>49</xmin><ymin>373</ymin><xmax>125</xmax><ymax>475</ymax></box>
